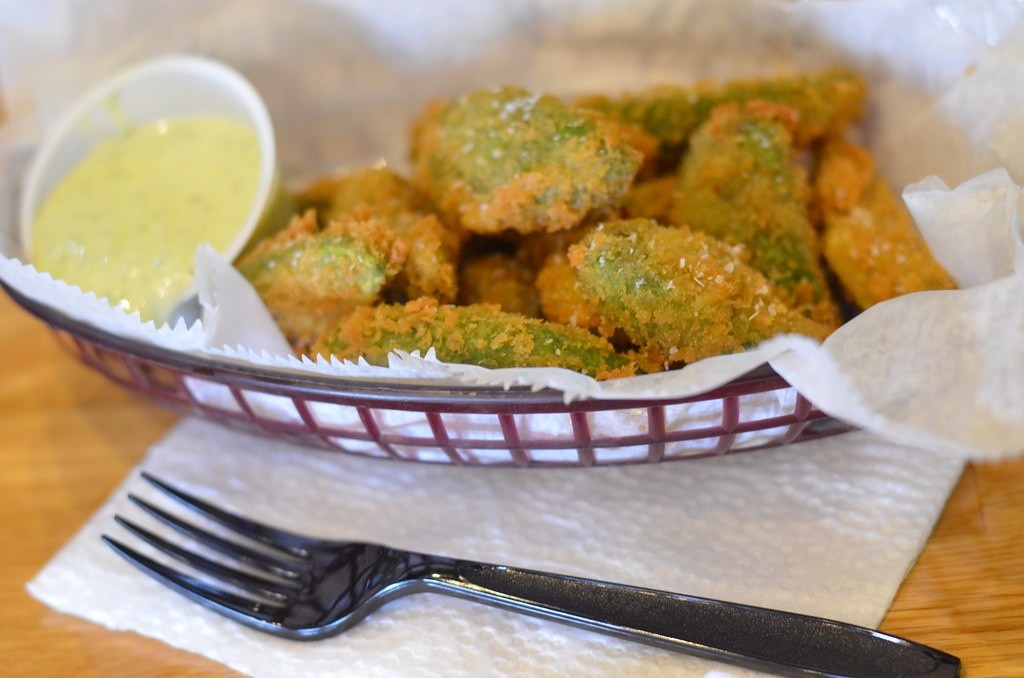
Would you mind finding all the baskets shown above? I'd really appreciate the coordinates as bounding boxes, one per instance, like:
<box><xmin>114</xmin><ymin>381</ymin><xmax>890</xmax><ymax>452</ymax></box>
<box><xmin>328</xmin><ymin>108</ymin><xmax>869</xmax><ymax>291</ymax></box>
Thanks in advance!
<box><xmin>0</xmin><ymin>280</ymin><xmax>866</xmax><ymax>468</ymax></box>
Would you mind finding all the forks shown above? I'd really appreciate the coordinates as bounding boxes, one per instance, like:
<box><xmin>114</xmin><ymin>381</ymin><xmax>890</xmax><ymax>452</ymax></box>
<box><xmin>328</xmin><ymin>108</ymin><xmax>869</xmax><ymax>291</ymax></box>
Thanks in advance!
<box><xmin>102</xmin><ymin>470</ymin><xmax>961</xmax><ymax>678</ymax></box>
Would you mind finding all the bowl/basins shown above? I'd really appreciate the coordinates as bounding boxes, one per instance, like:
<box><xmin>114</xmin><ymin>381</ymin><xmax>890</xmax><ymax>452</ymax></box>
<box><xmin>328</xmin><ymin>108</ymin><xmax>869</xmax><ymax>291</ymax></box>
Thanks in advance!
<box><xmin>12</xmin><ymin>51</ymin><xmax>297</xmax><ymax>329</ymax></box>
